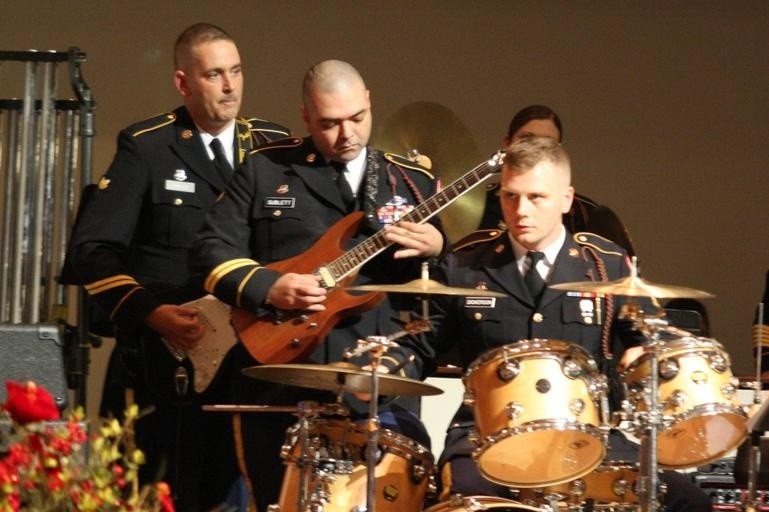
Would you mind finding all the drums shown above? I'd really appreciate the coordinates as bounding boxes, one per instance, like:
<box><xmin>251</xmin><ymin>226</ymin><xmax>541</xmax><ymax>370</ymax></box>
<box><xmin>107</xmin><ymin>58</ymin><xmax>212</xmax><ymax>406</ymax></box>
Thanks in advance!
<box><xmin>516</xmin><ymin>460</ymin><xmax>642</xmax><ymax>511</ymax></box>
<box><xmin>462</xmin><ymin>338</ymin><xmax>610</xmax><ymax>487</ymax></box>
<box><xmin>298</xmin><ymin>415</ymin><xmax>433</xmax><ymax>512</ymax></box>
<box><xmin>428</xmin><ymin>495</ymin><xmax>541</xmax><ymax>511</ymax></box>
<box><xmin>620</xmin><ymin>338</ymin><xmax>747</xmax><ymax>468</ymax></box>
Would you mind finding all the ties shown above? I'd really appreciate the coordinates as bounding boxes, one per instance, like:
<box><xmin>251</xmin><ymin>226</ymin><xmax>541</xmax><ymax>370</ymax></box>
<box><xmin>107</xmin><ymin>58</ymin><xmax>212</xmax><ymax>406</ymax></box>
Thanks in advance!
<box><xmin>331</xmin><ymin>160</ymin><xmax>352</xmax><ymax>203</ymax></box>
<box><xmin>209</xmin><ymin>138</ymin><xmax>232</xmax><ymax>171</ymax></box>
<box><xmin>524</xmin><ymin>251</ymin><xmax>547</xmax><ymax>296</ymax></box>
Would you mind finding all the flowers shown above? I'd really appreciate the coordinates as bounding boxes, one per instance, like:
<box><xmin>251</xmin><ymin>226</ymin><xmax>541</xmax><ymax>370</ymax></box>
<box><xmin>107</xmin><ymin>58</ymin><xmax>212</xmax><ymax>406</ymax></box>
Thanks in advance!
<box><xmin>0</xmin><ymin>376</ymin><xmax>180</xmax><ymax>512</ymax></box>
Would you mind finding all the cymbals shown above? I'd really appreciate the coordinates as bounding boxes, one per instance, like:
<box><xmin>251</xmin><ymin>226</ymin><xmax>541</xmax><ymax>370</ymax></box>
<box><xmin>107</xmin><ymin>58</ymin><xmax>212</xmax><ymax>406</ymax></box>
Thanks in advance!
<box><xmin>549</xmin><ymin>277</ymin><xmax>717</xmax><ymax>301</ymax></box>
<box><xmin>345</xmin><ymin>278</ymin><xmax>507</xmax><ymax>297</ymax></box>
<box><xmin>243</xmin><ymin>363</ymin><xmax>445</xmax><ymax>396</ymax></box>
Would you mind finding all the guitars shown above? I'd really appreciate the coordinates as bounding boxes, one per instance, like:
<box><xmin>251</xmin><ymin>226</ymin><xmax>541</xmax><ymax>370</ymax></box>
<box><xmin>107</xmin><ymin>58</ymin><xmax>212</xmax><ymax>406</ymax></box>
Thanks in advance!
<box><xmin>231</xmin><ymin>150</ymin><xmax>513</xmax><ymax>364</ymax></box>
<box><xmin>156</xmin><ymin>293</ymin><xmax>236</xmax><ymax>394</ymax></box>
